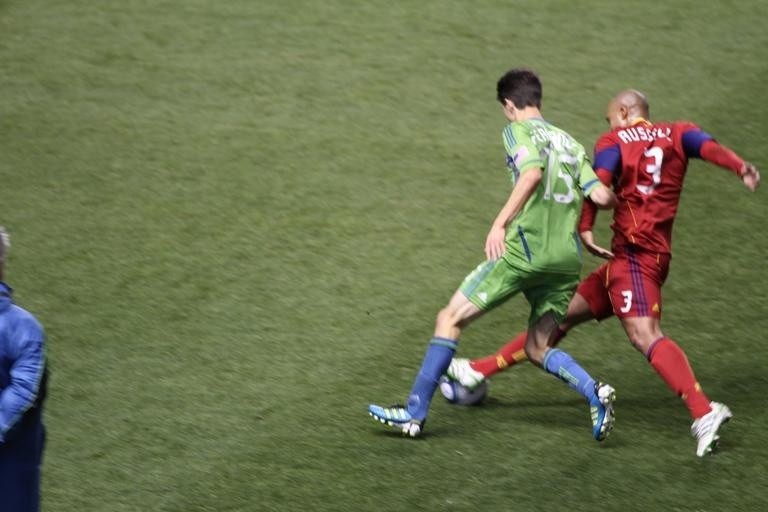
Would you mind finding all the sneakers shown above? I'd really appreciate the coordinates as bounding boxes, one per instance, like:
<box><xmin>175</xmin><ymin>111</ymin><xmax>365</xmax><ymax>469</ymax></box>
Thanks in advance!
<box><xmin>446</xmin><ymin>357</ymin><xmax>485</xmax><ymax>391</ymax></box>
<box><xmin>589</xmin><ymin>381</ymin><xmax>616</xmax><ymax>441</ymax></box>
<box><xmin>368</xmin><ymin>404</ymin><xmax>426</xmax><ymax>438</ymax></box>
<box><xmin>690</xmin><ymin>401</ymin><xmax>733</xmax><ymax>458</ymax></box>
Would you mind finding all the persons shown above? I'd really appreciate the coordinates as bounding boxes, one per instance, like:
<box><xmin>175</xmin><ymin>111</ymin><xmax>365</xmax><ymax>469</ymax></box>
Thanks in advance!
<box><xmin>368</xmin><ymin>70</ymin><xmax>619</xmax><ymax>442</ymax></box>
<box><xmin>450</xmin><ymin>88</ymin><xmax>759</xmax><ymax>456</ymax></box>
<box><xmin>0</xmin><ymin>226</ymin><xmax>49</xmax><ymax>511</ymax></box>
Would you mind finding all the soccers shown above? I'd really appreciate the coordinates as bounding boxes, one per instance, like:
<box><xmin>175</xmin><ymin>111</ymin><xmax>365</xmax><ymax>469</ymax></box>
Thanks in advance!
<box><xmin>441</xmin><ymin>359</ymin><xmax>488</xmax><ymax>405</ymax></box>
